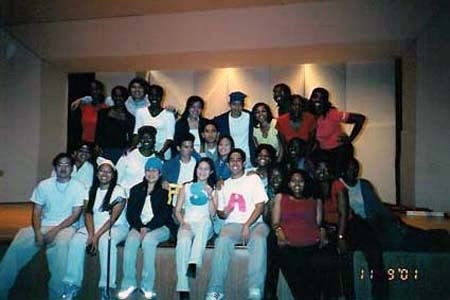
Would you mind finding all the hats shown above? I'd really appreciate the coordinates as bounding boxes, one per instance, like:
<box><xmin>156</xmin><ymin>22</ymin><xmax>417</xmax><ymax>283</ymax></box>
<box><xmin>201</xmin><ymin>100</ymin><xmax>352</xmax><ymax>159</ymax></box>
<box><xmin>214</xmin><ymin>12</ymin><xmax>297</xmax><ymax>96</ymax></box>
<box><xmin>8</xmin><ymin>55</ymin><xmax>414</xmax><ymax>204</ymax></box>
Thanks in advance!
<box><xmin>144</xmin><ymin>156</ymin><xmax>163</xmax><ymax>175</ymax></box>
<box><xmin>229</xmin><ymin>92</ymin><xmax>247</xmax><ymax>103</ymax></box>
<box><xmin>97</xmin><ymin>156</ymin><xmax>116</xmax><ymax>170</ymax></box>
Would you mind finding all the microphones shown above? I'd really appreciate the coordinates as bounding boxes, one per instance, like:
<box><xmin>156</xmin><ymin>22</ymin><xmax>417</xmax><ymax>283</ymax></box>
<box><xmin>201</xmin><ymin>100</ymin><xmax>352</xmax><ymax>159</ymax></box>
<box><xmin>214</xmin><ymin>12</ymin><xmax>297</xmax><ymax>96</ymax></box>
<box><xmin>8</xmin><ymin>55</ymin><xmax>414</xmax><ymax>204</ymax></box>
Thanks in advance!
<box><xmin>106</xmin><ymin>196</ymin><xmax>123</xmax><ymax>211</ymax></box>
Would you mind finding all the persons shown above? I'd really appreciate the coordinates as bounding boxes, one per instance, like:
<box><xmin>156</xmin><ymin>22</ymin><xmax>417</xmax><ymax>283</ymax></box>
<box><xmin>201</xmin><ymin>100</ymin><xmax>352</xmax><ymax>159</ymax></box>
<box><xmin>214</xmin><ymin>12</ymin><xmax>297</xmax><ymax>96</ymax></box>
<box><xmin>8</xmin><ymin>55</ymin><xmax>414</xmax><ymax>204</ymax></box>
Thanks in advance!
<box><xmin>52</xmin><ymin>77</ymin><xmax>399</xmax><ymax>300</ymax></box>
<box><xmin>0</xmin><ymin>153</ymin><xmax>85</xmax><ymax>300</ymax></box>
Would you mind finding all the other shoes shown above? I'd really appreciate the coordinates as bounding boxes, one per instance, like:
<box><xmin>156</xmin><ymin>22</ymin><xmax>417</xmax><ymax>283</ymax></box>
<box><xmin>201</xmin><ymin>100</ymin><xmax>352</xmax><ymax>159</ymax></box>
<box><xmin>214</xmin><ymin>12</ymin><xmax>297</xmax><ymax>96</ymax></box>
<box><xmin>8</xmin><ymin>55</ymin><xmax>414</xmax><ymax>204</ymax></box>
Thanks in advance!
<box><xmin>60</xmin><ymin>286</ymin><xmax>79</xmax><ymax>300</ymax></box>
<box><xmin>179</xmin><ymin>291</ymin><xmax>190</xmax><ymax>300</ymax></box>
<box><xmin>138</xmin><ymin>288</ymin><xmax>157</xmax><ymax>299</ymax></box>
<box><xmin>248</xmin><ymin>287</ymin><xmax>261</xmax><ymax>300</ymax></box>
<box><xmin>118</xmin><ymin>286</ymin><xmax>138</xmax><ymax>300</ymax></box>
<box><xmin>186</xmin><ymin>264</ymin><xmax>198</xmax><ymax>278</ymax></box>
<box><xmin>205</xmin><ymin>292</ymin><xmax>224</xmax><ymax>300</ymax></box>
<box><xmin>100</xmin><ymin>288</ymin><xmax>113</xmax><ymax>300</ymax></box>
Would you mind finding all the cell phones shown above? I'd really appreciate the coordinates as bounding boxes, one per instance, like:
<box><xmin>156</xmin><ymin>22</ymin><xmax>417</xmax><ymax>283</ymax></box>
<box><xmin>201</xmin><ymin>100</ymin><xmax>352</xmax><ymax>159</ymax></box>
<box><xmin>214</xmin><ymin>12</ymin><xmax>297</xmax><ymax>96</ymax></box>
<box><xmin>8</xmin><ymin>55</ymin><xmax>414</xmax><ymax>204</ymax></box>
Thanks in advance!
<box><xmin>86</xmin><ymin>244</ymin><xmax>97</xmax><ymax>257</ymax></box>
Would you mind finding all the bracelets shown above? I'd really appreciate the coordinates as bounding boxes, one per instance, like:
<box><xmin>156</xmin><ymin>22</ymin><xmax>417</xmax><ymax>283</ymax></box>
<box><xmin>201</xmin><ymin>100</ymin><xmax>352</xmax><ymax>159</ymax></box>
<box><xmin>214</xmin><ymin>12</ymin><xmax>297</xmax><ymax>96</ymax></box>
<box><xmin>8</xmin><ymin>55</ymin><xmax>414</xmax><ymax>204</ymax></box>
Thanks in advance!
<box><xmin>338</xmin><ymin>234</ymin><xmax>344</xmax><ymax>239</ymax></box>
<box><xmin>273</xmin><ymin>223</ymin><xmax>282</xmax><ymax>230</ymax></box>
